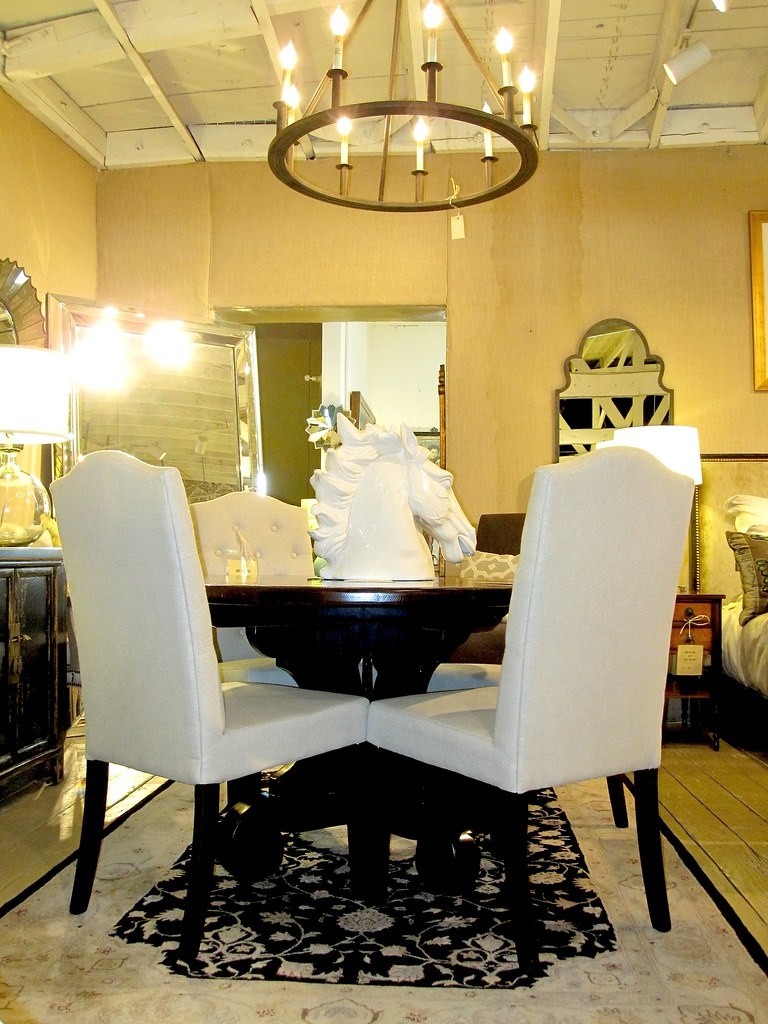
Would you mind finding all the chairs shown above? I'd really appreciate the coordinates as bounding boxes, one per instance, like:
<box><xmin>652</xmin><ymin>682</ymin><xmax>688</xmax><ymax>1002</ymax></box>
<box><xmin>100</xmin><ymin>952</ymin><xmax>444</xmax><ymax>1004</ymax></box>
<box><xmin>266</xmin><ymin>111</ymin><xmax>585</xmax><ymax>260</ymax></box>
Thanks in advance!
<box><xmin>367</xmin><ymin>447</ymin><xmax>694</xmax><ymax>978</ymax></box>
<box><xmin>194</xmin><ymin>493</ymin><xmax>321</xmax><ymax>808</ymax></box>
<box><xmin>52</xmin><ymin>450</ymin><xmax>373</xmax><ymax>963</ymax></box>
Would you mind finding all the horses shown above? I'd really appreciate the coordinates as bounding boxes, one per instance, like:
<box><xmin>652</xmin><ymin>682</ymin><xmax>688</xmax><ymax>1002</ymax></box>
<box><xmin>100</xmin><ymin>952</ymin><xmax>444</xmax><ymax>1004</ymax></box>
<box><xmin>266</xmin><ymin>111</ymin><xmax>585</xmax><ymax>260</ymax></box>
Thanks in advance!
<box><xmin>306</xmin><ymin>412</ymin><xmax>477</xmax><ymax>582</ymax></box>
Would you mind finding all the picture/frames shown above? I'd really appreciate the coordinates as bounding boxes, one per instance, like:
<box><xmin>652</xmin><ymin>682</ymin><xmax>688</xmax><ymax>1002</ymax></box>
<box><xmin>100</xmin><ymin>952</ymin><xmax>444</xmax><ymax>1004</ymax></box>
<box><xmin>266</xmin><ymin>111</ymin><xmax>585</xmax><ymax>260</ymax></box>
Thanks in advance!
<box><xmin>748</xmin><ymin>212</ymin><xmax>768</xmax><ymax>390</ymax></box>
<box><xmin>46</xmin><ymin>293</ymin><xmax>261</xmax><ymax>502</ymax></box>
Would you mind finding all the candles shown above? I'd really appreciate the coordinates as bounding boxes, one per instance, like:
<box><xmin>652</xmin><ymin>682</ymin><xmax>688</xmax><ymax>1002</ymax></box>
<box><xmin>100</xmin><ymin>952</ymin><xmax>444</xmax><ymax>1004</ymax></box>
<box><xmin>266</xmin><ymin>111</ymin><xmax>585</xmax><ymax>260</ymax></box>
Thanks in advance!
<box><xmin>278</xmin><ymin>4</ymin><xmax>536</xmax><ymax>171</ymax></box>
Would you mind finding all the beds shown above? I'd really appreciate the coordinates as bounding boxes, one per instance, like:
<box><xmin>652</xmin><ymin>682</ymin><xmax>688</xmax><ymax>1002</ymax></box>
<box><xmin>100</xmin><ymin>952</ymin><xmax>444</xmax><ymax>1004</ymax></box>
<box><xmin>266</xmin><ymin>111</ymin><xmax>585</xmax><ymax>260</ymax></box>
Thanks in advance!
<box><xmin>693</xmin><ymin>453</ymin><xmax>768</xmax><ymax>702</ymax></box>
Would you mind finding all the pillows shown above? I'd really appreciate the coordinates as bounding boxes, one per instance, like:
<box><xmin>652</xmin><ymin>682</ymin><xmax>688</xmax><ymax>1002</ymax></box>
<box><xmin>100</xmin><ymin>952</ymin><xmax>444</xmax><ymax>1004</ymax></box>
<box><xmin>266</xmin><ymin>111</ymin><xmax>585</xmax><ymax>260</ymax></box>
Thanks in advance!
<box><xmin>460</xmin><ymin>549</ymin><xmax>521</xmax><ymax>621</ymax></box>
<box><xmin>727</xmin><ymin>497</ymin><xmax>768</xmax><ymax>534</ymax></box>
<box><xmin>727</xmin><ymin>530</ymin><xmax>768</xmax><ymax>625</ymax></box>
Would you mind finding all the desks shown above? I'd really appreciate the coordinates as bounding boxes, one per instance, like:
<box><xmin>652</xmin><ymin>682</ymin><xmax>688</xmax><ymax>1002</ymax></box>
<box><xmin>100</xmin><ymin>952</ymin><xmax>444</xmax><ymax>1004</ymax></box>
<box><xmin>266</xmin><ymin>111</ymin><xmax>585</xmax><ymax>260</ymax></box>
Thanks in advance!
<box><xmin>204</xmin><ymin>580</ymin><xmax>511</xmax><ymax>901</ymax></box>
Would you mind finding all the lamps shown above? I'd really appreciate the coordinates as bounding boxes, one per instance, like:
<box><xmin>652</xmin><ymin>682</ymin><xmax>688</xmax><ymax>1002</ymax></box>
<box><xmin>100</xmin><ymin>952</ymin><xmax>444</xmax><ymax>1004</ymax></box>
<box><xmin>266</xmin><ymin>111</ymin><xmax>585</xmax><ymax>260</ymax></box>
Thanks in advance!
<box><xmin>614</xmin><ymin>427</ymin><xmax>702</xmax><ymax>594</ymax></box>
<box><xmin>0</xmin><ymin>344</ymin><xmax>66</xmax><ymax>545</ymax></box>
<box><xmin>268</xmin><ymin>0</ymin><xmax>537</xmax><ymax>211</ymax></box>
<box><xmin>663</xmin><ymin>41</ymin><xmax>713</xmax><ymax>86</ymax></box>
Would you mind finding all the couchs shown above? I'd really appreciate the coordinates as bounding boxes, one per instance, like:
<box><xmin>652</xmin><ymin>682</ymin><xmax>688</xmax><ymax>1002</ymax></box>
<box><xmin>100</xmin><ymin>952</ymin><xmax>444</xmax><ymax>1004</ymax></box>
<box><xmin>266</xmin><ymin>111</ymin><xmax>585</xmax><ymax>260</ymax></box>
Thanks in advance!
<box><xmin>443</xmin><ymin>513</ymin><xmax>529</xmax><ymax>663</ymax></box>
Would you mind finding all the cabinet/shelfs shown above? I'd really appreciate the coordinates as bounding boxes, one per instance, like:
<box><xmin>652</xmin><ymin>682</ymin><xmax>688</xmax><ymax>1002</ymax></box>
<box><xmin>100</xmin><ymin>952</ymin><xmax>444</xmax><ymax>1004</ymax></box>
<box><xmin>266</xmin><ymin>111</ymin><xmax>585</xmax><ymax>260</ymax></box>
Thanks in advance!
<box><xmin>1</xmin><ymin>546</ymin><xmax>64</xmax><ymax>806</ymax></box>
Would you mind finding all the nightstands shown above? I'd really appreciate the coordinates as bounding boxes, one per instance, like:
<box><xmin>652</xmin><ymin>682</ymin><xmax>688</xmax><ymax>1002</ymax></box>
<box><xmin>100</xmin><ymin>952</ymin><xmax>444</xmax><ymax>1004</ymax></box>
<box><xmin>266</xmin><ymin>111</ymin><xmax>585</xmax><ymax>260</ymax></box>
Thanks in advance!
<box><xmin>661</xmin><ymin>595</ymin><xmax>718</xmax><ymax>753</ymax></box>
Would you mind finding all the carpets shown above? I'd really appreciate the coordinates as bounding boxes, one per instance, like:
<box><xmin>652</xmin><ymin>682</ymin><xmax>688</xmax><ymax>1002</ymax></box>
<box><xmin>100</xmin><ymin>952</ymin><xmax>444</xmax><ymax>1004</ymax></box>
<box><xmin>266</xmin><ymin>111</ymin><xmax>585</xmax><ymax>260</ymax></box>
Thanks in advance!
<box><xmin>3</xmin><ymin>777</ymin><xmax>768</xmax><ymax>1023</ymax></box>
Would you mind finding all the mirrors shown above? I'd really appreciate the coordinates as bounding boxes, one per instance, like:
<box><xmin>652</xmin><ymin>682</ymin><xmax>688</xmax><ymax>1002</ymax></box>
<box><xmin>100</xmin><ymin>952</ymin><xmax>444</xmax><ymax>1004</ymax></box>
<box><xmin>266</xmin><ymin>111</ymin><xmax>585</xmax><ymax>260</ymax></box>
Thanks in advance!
<box><xmin>552</xmin><ymin>318</ymin><xmax>672</xmax><ymax>462</ymax></box>
